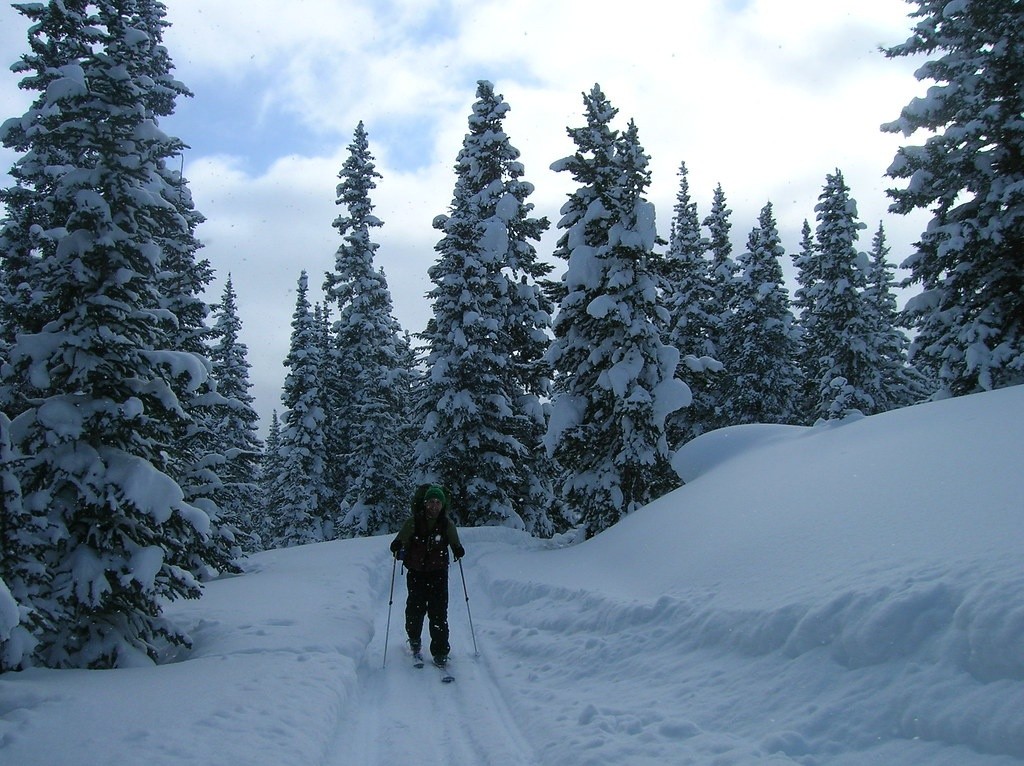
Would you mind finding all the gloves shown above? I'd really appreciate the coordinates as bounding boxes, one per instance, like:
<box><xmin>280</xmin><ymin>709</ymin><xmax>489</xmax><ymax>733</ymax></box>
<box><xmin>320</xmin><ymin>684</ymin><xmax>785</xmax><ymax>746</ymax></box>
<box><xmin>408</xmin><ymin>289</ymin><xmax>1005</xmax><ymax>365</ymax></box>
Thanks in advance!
<box><xmin>453</xmin><ymin>547</ymin><xmax>465</xmax><ymax>562</ymax></box>
<box><xmin>395</xmin><ymin>548</ymin><xmax>408</xmax><ymax>560</ymax></box>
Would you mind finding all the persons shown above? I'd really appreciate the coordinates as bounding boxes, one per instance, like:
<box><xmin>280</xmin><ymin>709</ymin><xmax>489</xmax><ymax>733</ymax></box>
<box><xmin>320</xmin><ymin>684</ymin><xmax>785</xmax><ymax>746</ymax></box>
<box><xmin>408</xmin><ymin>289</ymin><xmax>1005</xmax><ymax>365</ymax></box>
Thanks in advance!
<box><xmin>391</xmin><ymin>488</ymin><xmax>466</xmax><ymax>668</ymax></box>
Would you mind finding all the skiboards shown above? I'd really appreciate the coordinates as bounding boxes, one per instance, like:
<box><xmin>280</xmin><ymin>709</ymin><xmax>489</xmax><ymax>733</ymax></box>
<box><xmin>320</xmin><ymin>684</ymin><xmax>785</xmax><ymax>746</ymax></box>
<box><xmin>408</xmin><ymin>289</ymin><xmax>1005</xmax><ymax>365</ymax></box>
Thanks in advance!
<box><xmin>406</xmin><ymin>639</ymin><xmax>424</xmax><ymax>668</ymax></box>
<box><xmin>432</xmin><ymin>658</ymin><xmax>456</xmax><ymax>684</ymax></box>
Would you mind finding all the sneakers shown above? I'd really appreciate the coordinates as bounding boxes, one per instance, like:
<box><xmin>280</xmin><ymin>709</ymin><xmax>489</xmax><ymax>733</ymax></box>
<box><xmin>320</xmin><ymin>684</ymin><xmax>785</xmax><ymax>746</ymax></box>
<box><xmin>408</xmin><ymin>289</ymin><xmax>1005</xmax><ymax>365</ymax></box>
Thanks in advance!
<box><xmin>408</xmin><ymin>637</ymin><xmax>422</xmax><ymax>652</ymax></box>
<box><xmin>434</xmin><ymin>654</ymin><xmax>448</xmax><ymax>664</ymax></box>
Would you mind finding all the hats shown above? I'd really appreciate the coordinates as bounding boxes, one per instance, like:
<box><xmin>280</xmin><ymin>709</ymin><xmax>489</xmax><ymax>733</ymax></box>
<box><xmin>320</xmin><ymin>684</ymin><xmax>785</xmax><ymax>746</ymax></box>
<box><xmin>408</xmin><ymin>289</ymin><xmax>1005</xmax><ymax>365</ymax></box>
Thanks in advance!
<box><xmin>423</xmin><ymin>485</ymin><xmax>446</xmax><ymax>508</ymax></box>
